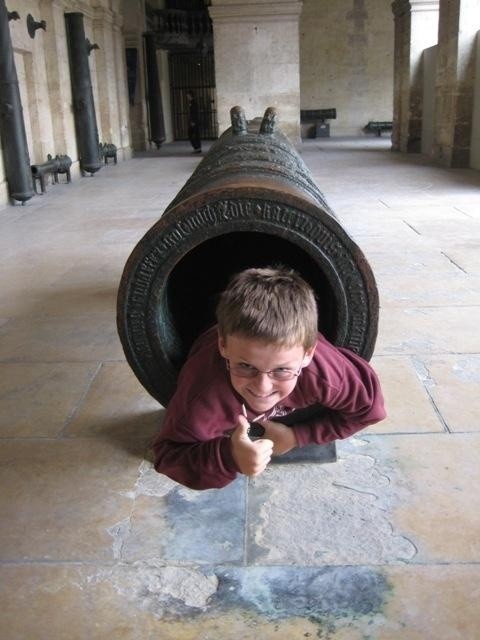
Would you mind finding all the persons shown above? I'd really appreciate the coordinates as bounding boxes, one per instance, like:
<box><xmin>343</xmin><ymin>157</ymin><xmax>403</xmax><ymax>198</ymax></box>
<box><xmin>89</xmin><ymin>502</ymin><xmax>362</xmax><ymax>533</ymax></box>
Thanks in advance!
<box><xmin>151</xmin><ymin>265</ymin><xmax>387</xmax><ymax>491</ymax></box>
<box><xmin>186</xmin><ymin>91</ymin><xmax>202</xmax><ymax>154</ymax></box>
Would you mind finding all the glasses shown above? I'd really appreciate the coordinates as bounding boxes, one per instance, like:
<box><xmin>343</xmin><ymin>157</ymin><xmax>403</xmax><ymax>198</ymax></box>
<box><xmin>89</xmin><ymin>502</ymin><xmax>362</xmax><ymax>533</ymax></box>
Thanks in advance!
<box><xmin>226</xmin><ymin>351</ymin><xmax>308</xmax><ymax>381</ymax></box>
